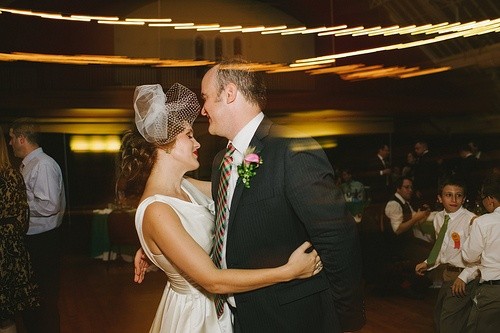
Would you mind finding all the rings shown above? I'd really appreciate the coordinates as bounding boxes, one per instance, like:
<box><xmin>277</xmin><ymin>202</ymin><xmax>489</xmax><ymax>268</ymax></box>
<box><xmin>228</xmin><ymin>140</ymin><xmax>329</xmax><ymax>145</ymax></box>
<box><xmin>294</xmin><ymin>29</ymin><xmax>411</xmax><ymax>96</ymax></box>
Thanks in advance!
<box><xmin>316</xmin><ymin>264</ymin><xmax>319</xmax><ymax>268</ymax></box>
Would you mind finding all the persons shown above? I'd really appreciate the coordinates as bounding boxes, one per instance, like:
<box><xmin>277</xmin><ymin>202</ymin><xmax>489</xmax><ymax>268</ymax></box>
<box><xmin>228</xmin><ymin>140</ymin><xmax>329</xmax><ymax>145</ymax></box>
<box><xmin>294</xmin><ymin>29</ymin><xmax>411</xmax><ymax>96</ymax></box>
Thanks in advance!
<box><xmin>461</xmin><ymin>177</ymin><xmax>500</xmax><ymax>333</ymax></box>
<box><xmin>415</xmin><ymin>175</ymin><xmax>484</xmax><ymax>333</ymax></box>
<box><xmin>407</xmin><ymin>138</ymin><xmax>440</xmax><ymax>203</ymax></box>
<box><xmin>451</xmin><ymin>141</ymin><xmax>489</xmax><ymax>214</ymax></box>
<box><xmin>401</xmin><ymin>152</ymin><xmax>416</xmax><ymax>184</ymax></box>
<box><xmin>116</xmin><ymin>81</ymin><xmax>323</xmax><ymax>333</ymax></box>
<box><xmin>465</xmin><ymin>140</ymin><xmax>500</xmax><ymax>189</ymax></box>
<box><xmin>419</xmin><ymin>144</ymin><xmax>482</xmax><ymax>224</ymax></box>
<box><xmin>338</xmin><ymin>169</ymin><xmax>367</xmax><ymax>233</ymax></box>
<box><xmin>381</xmin><ymin>177</ymin><xmax>434</xmax><ymax>295</ymax></box>
<box><xmin>131</xmin><ymin>58</ymin><xmax>366</xmax><ymax>333</ymax></box>
<box><xmin>8</xmin><ymin>117</ymin><xmax>66</xmax><ymax>332</ymax></box>
<box><xmin>0</xmin><ymin>126</ymin><xmax>41</xmax><ymax>333</ymax></box>
<box><xmin>366</xmin><ymin>141</ymin><xmax>395</xmax><ymax>240</ymax></box>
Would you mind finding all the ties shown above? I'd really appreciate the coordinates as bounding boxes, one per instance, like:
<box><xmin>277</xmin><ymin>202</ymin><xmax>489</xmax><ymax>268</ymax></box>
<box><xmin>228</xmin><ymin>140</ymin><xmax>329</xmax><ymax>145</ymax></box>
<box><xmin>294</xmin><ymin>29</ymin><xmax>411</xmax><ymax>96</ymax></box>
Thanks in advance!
<box><xmin>212</xmin><ymin>143</ymin><xmax>236</xmax><ymax>319</ymax></box>
<box><xmin>427</xmin><ymin>215</ymin><xmax>450</xmax><ymax>265</ymax></box>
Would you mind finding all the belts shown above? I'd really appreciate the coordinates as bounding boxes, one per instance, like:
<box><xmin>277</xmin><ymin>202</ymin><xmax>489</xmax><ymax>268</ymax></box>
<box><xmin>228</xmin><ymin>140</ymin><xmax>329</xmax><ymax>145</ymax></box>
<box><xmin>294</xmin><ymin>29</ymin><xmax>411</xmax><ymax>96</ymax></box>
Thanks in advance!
<box><xmin>447</xmin><ymin>266</ymin><xmax>464</xmax><ymax>272</ymax></box>
<box><xmin>483</xmin><ymin>280</ymin><xmax>500</xmax><ymax>285</ymax></box>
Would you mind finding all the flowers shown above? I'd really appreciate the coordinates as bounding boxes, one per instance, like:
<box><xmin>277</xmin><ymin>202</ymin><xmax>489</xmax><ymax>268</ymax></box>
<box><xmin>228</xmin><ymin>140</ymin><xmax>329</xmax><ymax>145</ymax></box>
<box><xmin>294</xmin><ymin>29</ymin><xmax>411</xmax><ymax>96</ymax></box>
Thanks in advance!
<box><xmin>237</xmin><ymin>145</ymin><xmax>263</xmax><ymax>189</ymax></box>
